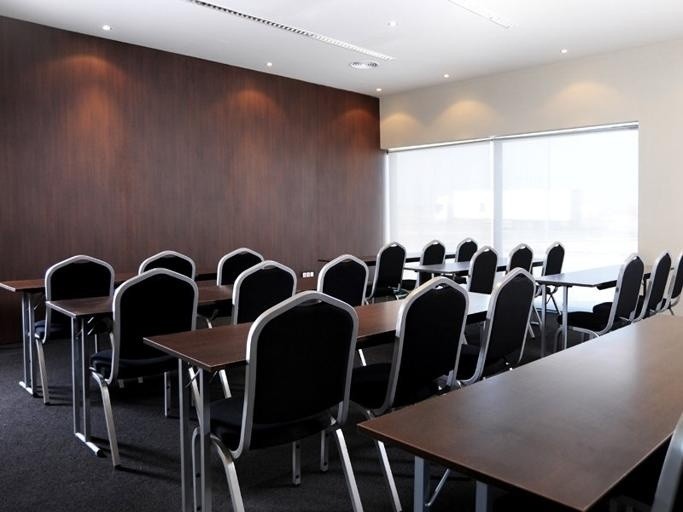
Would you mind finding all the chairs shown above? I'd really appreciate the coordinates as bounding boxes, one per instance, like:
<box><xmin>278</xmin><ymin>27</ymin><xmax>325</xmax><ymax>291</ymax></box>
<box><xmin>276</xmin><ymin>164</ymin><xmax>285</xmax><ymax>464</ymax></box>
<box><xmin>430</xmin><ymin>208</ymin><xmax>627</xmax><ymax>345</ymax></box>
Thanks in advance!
<box><xmin>553</xmin><ymin>256</ymin><xmax>644</xmax><ymax>353</ymax></box>
<box><xmin>661</xmin><ymin>256</ymin><xmax>683</xmax><ymax>311</ymax></box>
<box><xmin>594</xmin><ymin>252</ymin><xmax>671</xmax><ymax>322</ymax></box>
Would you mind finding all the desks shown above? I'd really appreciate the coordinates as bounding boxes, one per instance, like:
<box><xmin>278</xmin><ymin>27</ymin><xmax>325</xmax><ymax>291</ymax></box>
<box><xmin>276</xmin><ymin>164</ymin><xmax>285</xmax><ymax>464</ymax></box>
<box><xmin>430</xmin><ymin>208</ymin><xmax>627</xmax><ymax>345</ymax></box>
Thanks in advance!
<box><xmin>535</xmin><ymin>265</ymin><xmax>674</xmax><ymax>358</ymax></box>
<box><xmin>356</xmin><ymin>299</ymin><xmax>683</xmax><ymax>512</ymax></box>
